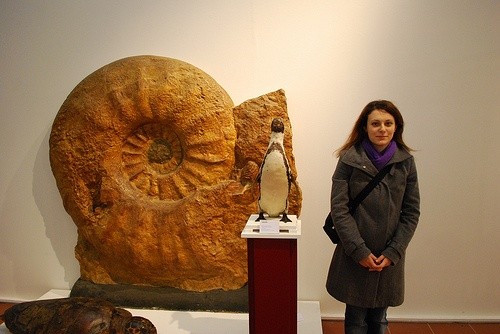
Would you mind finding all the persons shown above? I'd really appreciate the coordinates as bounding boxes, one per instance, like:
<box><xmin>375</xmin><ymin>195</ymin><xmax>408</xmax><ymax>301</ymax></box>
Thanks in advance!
<box><xmin>323</xmin><ymin>98</ymin><xmax>419</xmax><ymax>334</ymax></box>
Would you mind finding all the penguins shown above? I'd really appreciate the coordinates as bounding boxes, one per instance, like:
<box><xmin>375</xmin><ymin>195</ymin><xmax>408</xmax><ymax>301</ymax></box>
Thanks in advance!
<box><xmin>242</xmin><ymin>118</ymin><xmax>294</xmax><ymax>223</ymax></box>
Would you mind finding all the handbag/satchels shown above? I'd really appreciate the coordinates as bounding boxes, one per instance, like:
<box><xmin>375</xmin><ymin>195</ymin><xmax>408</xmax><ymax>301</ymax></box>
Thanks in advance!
<box><xmin>323</xmin><ymin>164</ymin><xmax>393</xmax><ymax>244</ymax></box>
